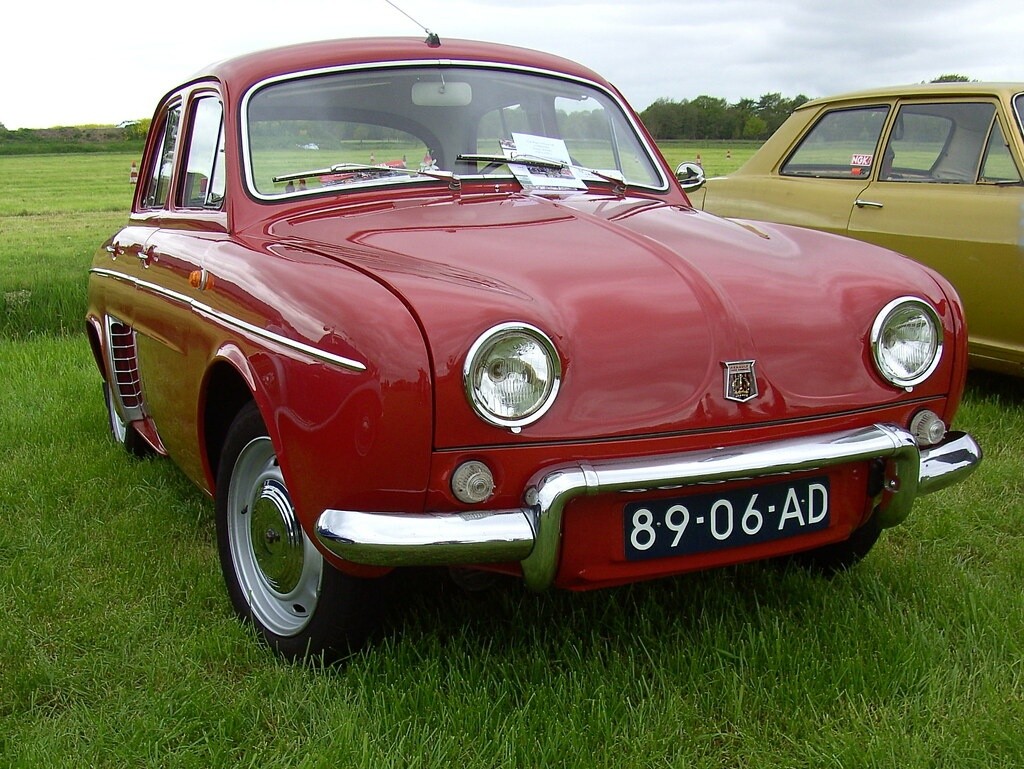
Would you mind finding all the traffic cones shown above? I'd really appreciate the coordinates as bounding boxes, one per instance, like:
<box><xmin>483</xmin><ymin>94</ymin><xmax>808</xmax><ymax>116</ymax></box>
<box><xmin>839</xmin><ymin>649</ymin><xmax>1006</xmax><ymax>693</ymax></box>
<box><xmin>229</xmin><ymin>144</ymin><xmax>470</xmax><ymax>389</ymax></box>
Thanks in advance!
<box><xmin>696</xmin><ymin>154</ymin><xmax>702</xmax><ymax>166</ymax></box>
<box><xmin>402</xmin><ymin>155</ymin><xmax>407</xmax><ymax>168</ymax></box>
<box><xmin>726</xmin><ymin>150</ymin><xmax>731</xmax><ymax>160</ymax></box>
<box><xmin>128</xmin><ymin>161</ymin><xmax>139</xmax><ymax>184</ymax></box>
<box><xmin>370</xmin><ymin>153</ymin><xmax>374</xmax><ymax>165</ymax></box>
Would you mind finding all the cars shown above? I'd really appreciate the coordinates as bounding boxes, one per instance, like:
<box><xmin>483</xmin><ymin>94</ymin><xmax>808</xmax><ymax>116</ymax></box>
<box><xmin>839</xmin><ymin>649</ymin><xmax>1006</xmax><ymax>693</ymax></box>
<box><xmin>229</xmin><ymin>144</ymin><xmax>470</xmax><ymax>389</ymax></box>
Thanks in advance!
<box><xmin>83</xmin><ymin>0</ymin><xmax>982</xmax><ymax>669</ymax></box>
<box><xmin>686</xmin><ymin>81</ymin><xmax>1024</xmax><ymax>377</ymax></box>
